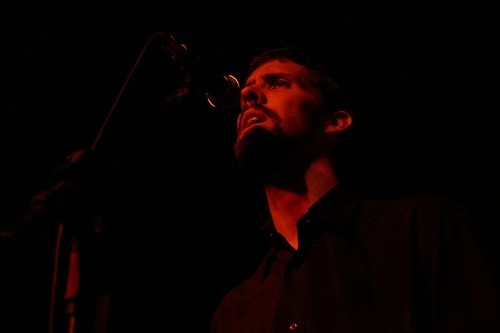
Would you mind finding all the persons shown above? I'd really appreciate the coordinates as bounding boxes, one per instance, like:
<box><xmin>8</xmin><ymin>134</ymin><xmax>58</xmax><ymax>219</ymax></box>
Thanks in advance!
<box><xmin>209</xmin><ymin>48</ymin><xmax>499</xmax><ymax>333</ymax></box>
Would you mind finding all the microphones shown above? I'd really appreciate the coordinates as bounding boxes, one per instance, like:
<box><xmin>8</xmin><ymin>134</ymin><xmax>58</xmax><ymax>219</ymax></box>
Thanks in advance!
<box><xmin>155</xmin><ymin>31</ymin><xmax>241</xmax><ymax>113</ymax></box>
